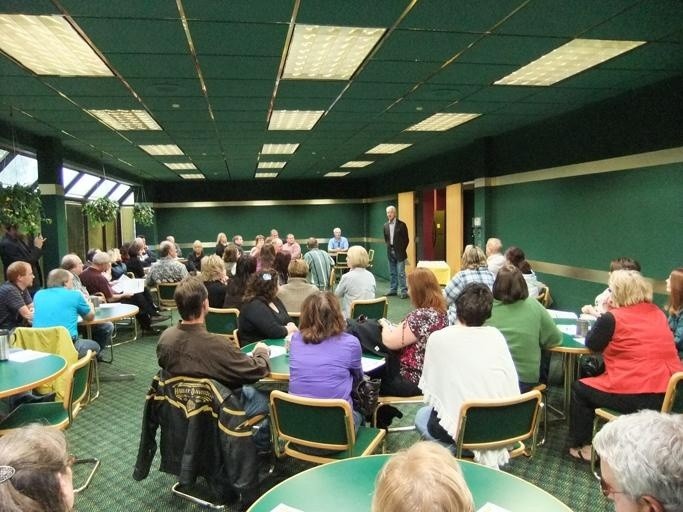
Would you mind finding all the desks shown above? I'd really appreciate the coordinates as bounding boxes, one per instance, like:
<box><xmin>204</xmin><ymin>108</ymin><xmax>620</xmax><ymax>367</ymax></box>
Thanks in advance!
<box><xmin>239</xmin><ymin>336</ymin><xmax>386</xmax><ymax>380</ymax></box>
<box><xmin>540</xmin><ymin>315</ymin><xmax>600</xmax><ymax>426</ymax></box>
<box><xmin>417</xmin><ymin>260</ymin><xmax>451</xmax><ymax>285</ymax></box>
<box><xmin>247</xmin><ymin>452</ymin><xmax>573</xmax><ymax>512</ymax></box>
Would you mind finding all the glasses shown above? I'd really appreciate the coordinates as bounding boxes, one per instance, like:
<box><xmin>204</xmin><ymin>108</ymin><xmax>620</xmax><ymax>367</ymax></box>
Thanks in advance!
<box><xmin>356</xmin><ymin>313</ymin><xmax>368</xmax><ymax>324</ymax></box>
<box><xmin>601</xmin><ymin>478</ymin><xmax>625</xmax><ymax>497</ymax></box>
<box><xmin>65</xmin><ymin>454</ymin><xmax>76</xmax><ymax>468</ymax></box>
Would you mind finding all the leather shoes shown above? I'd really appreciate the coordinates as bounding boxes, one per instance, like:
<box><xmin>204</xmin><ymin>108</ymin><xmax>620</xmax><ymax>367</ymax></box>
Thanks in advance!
<box><xmin>382</xmin><ymin>292</ymin><xmax>398</xmax><ymax>297</ymax></box>
<box><xmin>400</xmin><ymin>293</ymin><xmax>408</xmax><ymax>299</ymax></box>
<box><xmin>142</xmin><ymin>329</ymin><xmax>161</xmax><ymax>337</ymax></box>
<box><xmin>150</xmin><ymin>315</ymin><xmax>171</xmax><ymax>324</ymax></box>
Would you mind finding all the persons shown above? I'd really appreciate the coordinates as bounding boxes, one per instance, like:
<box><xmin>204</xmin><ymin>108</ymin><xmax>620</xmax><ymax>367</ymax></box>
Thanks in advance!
<box><xmin>591</xmin><ymin>409</ymin><xmax>683</xmax><ymax>512</ymax></box>
<box><xmin>380</xmin><ymin>237</ymin><xmax>563</xmax><ymax>459</ymax></box>
<box><xmin>372</xmin><ymin>441</ymin><xmax>475</xmax><ymax>512</ymax></box>
<box><xmin>563</xmin><ymin>257</ymin><xmax>683</xmax><ymax>506</ymax></box>
<box><xmin>156</xmin><ymin>269</ymin><xmax>363</xmax><ymax>456</ymax></box>
<box><xmin>382</xmin><ymin>206</ymin><xmax>409</xmax><ymax>299</ymax></box>
<box><xmin>1</xmin><ymin>221</ymin><xmax>157</xmax><ymax>358</ymax></box>
<box><xmin>158</xmin><ymin>227</ymin><xmax>375</xmax><ymax>323</ymax></box>
<box><xmin>0</xmin><ymin>422</ymin><xmax>74</xmax><ymax>512</ymax></box>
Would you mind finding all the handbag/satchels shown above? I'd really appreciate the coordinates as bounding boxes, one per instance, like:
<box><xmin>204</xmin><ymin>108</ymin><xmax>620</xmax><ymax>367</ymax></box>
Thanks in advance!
<box><xmin>350</xmin><ymin>378</ymin><xmax>382</xmax><ymax>416</ymax></box>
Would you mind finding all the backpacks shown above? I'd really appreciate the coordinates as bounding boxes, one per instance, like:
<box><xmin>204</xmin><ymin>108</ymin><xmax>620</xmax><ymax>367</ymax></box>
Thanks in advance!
<box><xmin>582</xmin><ymin>355</ymin><xmax>600</xmax><ymax>377</ymax></box>
<box><xmin>347</xmin><ymin>318</ymin><xmax>394</xmax><ymax>376</ymax></box>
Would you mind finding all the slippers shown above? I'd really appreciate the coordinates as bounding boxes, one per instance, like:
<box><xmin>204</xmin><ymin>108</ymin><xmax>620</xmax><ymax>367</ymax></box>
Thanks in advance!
<box><xmin>569</xmin><ymin>446</ymin><xmax>600</xmax><ymax>465</ymax></box>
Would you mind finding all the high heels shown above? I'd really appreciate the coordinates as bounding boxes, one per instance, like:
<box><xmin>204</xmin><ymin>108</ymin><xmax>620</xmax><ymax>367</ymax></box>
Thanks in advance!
<box><xmin>377</xmin><ymin>405</ymin><xmax>403</xmax><ymax>433</ymax></box>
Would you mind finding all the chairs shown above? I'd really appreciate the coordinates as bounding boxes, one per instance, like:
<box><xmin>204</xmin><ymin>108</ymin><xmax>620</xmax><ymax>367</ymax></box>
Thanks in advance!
<box><xmin>0</xmin><ymin>273</ymin><xmax>181</xmax><ymax>494</ymax></box>
<box><xmin>288</xmin><ymin>312</ymin><xmax>300</xmax><ymax>327</ymax></box>
<box><xmin>591</xmin><ymin>372</ymin><xmax>683</xmax><ymax>480</ymax></box>
<box><xmin>204</xmin><ymin>307</ymin><xmax>240</xmax><ymax>339</ymax></box>
<box><xmin>233</xmin><ymin>328</ymin><xmax>241</xmax><ymax>349</ymax></box>
<box><xmin>455</xmin><ymin>390</ymin><xmax>542</xmax><ymax>463</ymax></box>
<box><xmin>531</xmin><ymin>383</ymin><xmax>551</xmax><ymax>447</ymax></box>
<box><xmin>350</xmin><ymin>297</ymin><xmax>388</xmax><ymax>319</ymax></box>
<box><xmin>329</xmin><ymin>249</ymin><xmax>374</xmax><ymax>292</ymax></box>
<box><xmin>373</xmin><ymin>395</ymin><xmax>425</xmax><ymax>433</ymax></box>
<box><xmin>159</xmin><ymin>367</ymin><xmax>277</xmax><ymax>509</ymax></box>
<box><xmin>267</xmin><ymin>390</ymin><xmax>386</xmax><ymax>464</ymax></box>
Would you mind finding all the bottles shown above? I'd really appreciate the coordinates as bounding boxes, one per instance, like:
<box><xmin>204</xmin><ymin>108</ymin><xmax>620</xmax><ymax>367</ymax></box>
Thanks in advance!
<box><xmin>0</xmin><ymin>329</ymin><xmax>17</xmax><ymax>361</ymax></box>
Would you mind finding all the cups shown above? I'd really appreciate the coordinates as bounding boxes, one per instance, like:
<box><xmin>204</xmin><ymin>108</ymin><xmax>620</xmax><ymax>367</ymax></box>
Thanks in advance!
<box><xmin>575</xmin><ymin>319</ymin><xmax>588</xmax><ymax>337</ymax></box>
